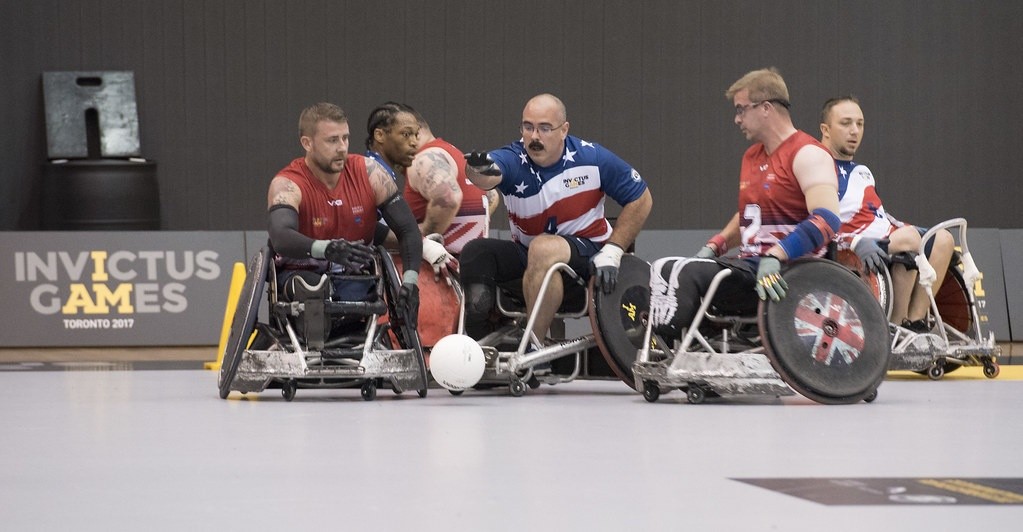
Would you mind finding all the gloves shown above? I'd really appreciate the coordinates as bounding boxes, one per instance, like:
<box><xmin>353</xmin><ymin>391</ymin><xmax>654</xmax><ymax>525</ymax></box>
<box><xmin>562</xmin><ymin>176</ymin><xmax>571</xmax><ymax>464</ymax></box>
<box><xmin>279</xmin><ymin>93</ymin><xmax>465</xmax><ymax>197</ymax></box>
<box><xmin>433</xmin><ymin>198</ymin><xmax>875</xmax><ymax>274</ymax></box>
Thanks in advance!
<box><xmin>396</xmin><ymin>269</ymin><xmax>420</xmax><ymax>330</ymax></box>
<box><xmin>311</xmin><ymin>237</ymin><xmax>376</xmax><ymax>275</ymax></box>
<box><xmin>755</xmin><ymin>253</ymin><xmax>789</xmax><ymax>302</ymax></box>
<box><xmin>587</xmin><ymin>242</ymin><xmax>624</xmax><ymax>294</ymax></box>
<box><xmin>849</xmin><ymin>234</ymin><xmax>892</xmax><ymax>276</ymax></box>
<box><xmin>420</xmin><ymin>237</ymin><xmax>461</xmax><ymax>286</ymax></box>
<box><xmin>464</xmin><ymin>150</ymin><xmax>502</xmax><ymax>177</ymax></box>
<box><xmin>691</xmin><ymin>248</ymin><xmax>715</xmax><ymax>260</ymax></box>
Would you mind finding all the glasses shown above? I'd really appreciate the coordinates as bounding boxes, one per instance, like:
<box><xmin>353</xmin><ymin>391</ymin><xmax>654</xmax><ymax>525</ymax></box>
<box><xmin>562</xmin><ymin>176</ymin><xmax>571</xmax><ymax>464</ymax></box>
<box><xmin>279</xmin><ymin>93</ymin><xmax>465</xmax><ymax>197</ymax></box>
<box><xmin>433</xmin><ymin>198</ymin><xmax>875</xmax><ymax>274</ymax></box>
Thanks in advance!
<box><xmin>733</xmin><ymin>98</ymin><xmax>773</xmax><ymax>119</ymax></box>
<box><xmin>518</xmin><ymin>119</ymin><xmax>566</xmax><ymax>138</ymax></box>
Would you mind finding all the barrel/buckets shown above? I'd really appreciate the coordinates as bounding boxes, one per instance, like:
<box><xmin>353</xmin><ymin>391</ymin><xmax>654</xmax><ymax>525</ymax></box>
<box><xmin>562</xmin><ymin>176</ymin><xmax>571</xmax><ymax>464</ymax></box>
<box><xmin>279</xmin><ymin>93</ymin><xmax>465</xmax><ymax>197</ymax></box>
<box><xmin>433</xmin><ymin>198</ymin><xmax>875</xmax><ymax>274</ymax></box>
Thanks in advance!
<box><xmin>42</xmin><ymin>158</ymin><xmax>162</xmax><ymax>231</ymax></box>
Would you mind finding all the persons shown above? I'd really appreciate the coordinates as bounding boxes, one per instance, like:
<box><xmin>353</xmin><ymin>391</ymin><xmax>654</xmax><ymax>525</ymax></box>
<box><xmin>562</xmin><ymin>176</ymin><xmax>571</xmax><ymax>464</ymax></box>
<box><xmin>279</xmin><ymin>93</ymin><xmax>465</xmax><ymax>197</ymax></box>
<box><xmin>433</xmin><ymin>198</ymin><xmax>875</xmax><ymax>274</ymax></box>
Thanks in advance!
<box><xmin>649</xmin><ymin>68</ymin><xmax>955</xmax><ymax>362</ymax></box>
<box><xmin>265</xmin><ymin>91</ymin><xmax>653</xmax><ymax>376</ymax></box>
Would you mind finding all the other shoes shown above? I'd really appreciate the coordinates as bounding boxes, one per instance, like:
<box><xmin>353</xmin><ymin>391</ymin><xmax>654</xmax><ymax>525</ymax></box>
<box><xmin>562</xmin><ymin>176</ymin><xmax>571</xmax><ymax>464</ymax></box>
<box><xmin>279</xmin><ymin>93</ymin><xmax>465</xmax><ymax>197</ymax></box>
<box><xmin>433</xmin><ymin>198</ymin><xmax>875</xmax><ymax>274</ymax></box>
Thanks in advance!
<box><xmin>515</xmin><ymin>341</ymin><xmax>552</xmax><ymax>377</ymax></box>
<box><xmin>892</xmin><ymin>320</ymin><xmax>931</xmax><ymax>347</ymax></box>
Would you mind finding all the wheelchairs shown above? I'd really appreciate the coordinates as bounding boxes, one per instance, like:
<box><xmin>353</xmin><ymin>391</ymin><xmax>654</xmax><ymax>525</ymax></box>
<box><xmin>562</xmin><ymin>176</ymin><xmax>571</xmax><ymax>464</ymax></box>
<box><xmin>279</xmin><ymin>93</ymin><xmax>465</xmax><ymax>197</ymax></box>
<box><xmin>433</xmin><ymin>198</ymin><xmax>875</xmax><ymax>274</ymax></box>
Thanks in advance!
<box><xmin>211</xmin><ymin>245</ymin><xmax>430</xmax><ymax>401</ymax></box>
<box><xmin>833</xmin><ymin>216</ymin><xmax>1003</xmax><ymax>381</ymax></box>
<box><xmin>378</xmin><ymin>249</ymin><xmax>465</xmax><ymax>381</ymax></box>
<box><xmin>631</xmin><ymin>257</ymin><xmax>891</xmax><ymax>405</ymax></box>
<box><xmin>444</xmin><ymin>250</ymin><xmax>651</xmax><ymax>397</ymax></box>
